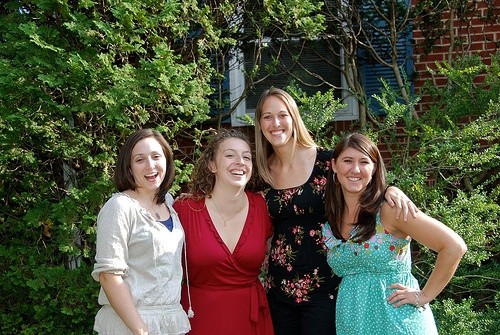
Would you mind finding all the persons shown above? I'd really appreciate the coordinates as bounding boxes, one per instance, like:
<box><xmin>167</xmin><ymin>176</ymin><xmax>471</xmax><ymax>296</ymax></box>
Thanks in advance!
<box><xmin>91</xmin><ymin>129</ymin><xmax>268</xmax><ymax>335</ymax></box>
<box><xmin>322</xmin><ymin>133</ymin><xmax>467</xmax><ymax>335</ymax></box>
<box><xmin>172</xmin><ymin>87</ymin><xmax>419</xmax><ymax>335</ymax></box>
<box><xmin>172</xmin><ymin>131</ymin><xmax>274</xmax><ymax>335</ymax></box>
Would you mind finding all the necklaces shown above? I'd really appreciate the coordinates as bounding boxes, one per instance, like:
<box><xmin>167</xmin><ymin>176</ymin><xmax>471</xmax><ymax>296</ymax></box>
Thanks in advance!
<box><xmin>141</xmin><ymin>202</ymin><xmax>161</xmax><ymax>220</ymax></box>
<box><xmin>212</xmin><ymin>196</ymin><xmax>245</xmax><ymax>227</ymax></box>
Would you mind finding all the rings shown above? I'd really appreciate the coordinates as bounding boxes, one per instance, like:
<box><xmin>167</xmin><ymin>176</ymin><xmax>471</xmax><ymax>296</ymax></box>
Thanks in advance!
<box><xmin>407</xmin><ymin>200</ymin><xmax>411</xmax><ymax>205</ymax></box>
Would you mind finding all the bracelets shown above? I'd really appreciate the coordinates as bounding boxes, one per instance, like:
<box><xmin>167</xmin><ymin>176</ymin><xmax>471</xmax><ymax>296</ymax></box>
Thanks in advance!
<box><xmin>414</xmin><ymin>289</ymin><xmax>421</xmax><ymax>308</ymax></box>
<box><xmin>383</xmin><ymin>185</ymin><xmax>390</xmax><ymax>202</ymax></box>
